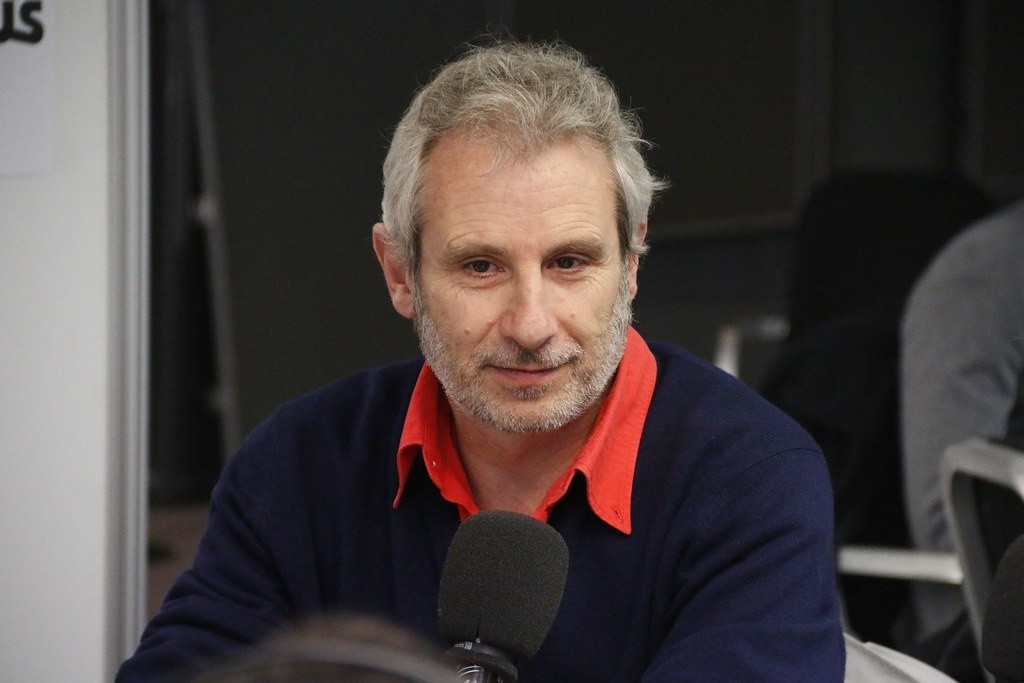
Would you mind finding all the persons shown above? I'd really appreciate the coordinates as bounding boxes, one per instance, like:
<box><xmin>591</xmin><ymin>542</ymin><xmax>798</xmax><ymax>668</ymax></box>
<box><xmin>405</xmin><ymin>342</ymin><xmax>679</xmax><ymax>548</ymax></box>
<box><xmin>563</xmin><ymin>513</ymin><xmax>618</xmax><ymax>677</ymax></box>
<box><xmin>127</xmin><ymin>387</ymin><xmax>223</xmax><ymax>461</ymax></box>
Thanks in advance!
<box><xmin>116</xmin><ymin>27</ymin><xmax>846</xmax><ymax>683</ymax></box>
<box><xmin>903</xmin><ymin>200</ymin><xmax>1024</xmax><ymax>683</ymax></box>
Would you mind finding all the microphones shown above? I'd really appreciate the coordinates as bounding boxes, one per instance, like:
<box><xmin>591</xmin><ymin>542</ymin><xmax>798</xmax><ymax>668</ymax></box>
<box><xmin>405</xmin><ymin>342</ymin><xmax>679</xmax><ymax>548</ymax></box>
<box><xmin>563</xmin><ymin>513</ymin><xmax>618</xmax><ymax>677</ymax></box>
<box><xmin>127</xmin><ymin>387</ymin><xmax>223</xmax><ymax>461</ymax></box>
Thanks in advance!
<box><xmin>437</xmin><ymin>511</ymin><xmax>569</xmax><ymax>683</ymax></box>
